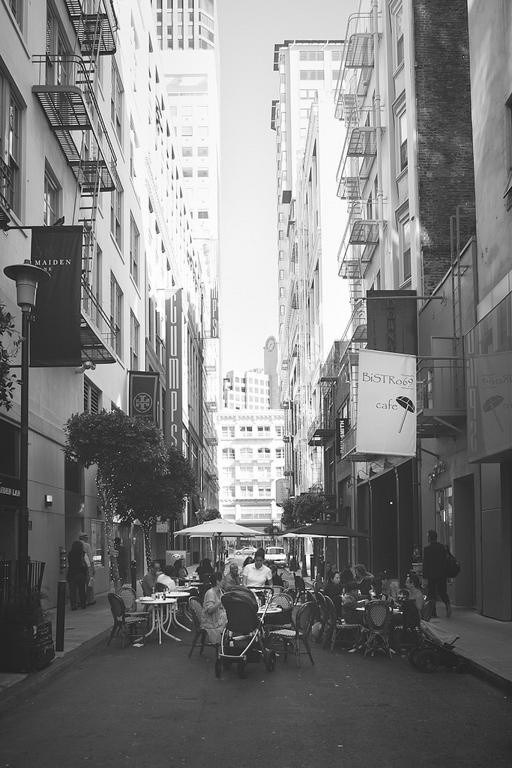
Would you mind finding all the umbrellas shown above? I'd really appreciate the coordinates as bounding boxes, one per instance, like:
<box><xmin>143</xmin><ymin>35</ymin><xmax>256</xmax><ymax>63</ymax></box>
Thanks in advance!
<box><xmin>173</xmin><ymin>519</ymin><xmax>264</xmax><ymax>570</ymax></box>
<box><xmin>295</xmin><ymin>514</ymin><xmax>372</xmax><ymax>582</ymax></box>
<box><xmin>279</xmin><ymin>532</ymin><xmax>350</xmax><ymax>538</ymax></box>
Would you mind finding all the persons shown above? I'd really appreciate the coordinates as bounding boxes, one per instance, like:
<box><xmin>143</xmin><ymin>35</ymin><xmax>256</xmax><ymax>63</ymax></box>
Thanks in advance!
<box><xmin>426</xmin><ymin>530</ymin><xmax>453</xmax><ymax>618</ymax></box>
<box><xmin>79</xmin><ymin>533</ymin><xmax>96</xmax><ymax>605</ymax></box>
<box><xmin>112</xmin><ymin>537</ymin><xmax>128</xmax><ymax>587</ymax></box>
<box><xmin>67</xmin><ymin>541</ymin><xmax>89</xmax><ymax>611</ymax></box>
<box><xmin>141</xmin><ymin>548</ymin><xmax>424</xmax><ymax>655</ymax></box>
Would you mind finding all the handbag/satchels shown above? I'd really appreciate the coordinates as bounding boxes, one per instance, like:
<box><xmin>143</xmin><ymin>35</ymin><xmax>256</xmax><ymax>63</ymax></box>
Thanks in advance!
<box><xmin>439</xmin><ymin>551</ymin><xmax>460</xmax><ymax>578</ymax></box>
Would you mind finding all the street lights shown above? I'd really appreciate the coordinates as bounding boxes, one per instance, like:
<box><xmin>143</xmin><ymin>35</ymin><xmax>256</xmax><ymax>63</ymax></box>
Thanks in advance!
<box><xmin>0</xmin><ymin>258</ymin><xmax>51</xmax><ymax>595</ymax></box>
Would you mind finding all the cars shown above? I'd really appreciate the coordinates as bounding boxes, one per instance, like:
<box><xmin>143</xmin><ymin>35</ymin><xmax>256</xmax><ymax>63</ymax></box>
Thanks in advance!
<box><xmin>234</xmin><ymin>545</ymin><xmax>257</xmax><ymax>555</ymax></box>
<box><xmin>264</xmin><ymin>545</ymin><xmax>287</xmax><ymax>567</ymax></box>
